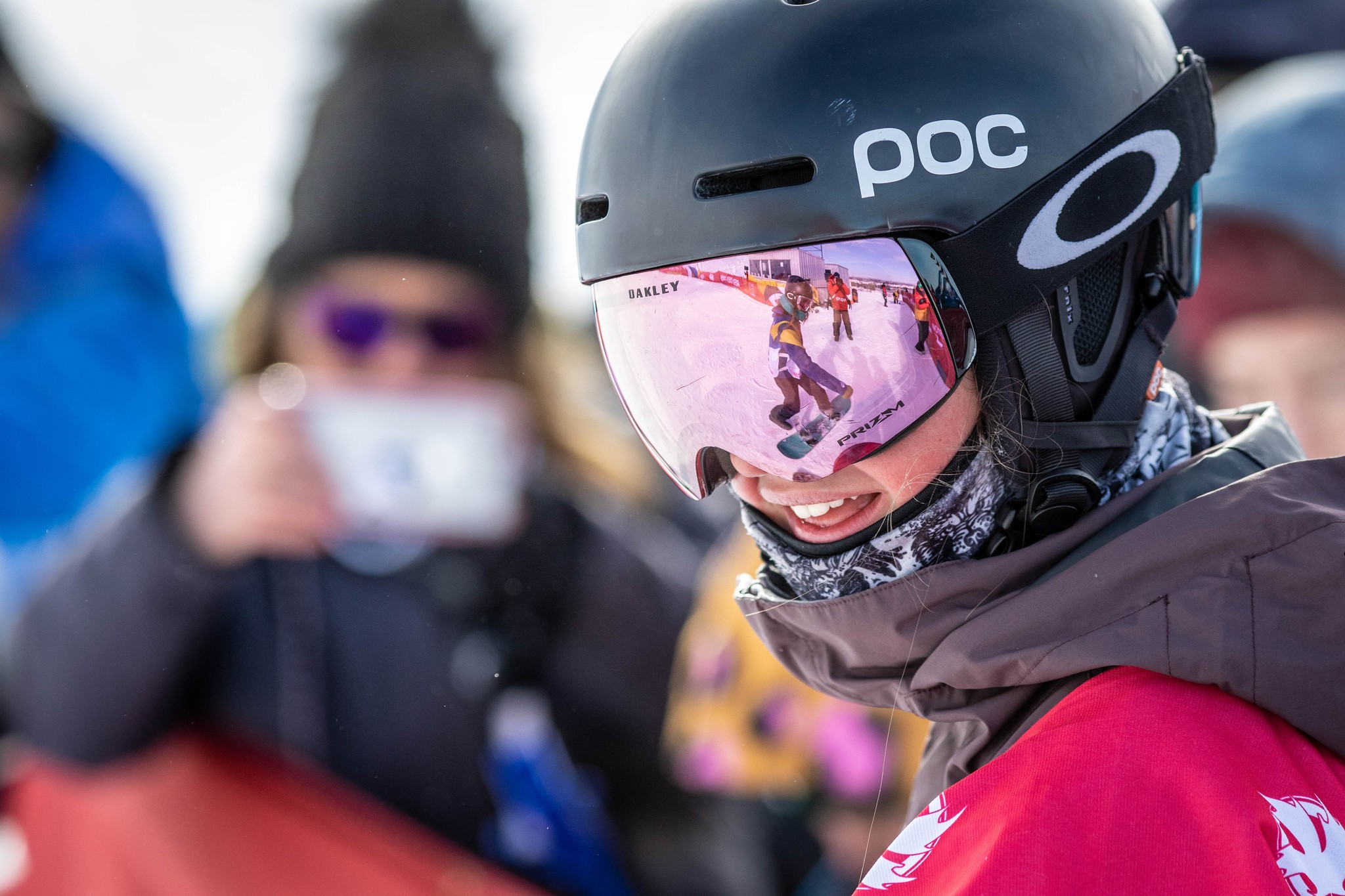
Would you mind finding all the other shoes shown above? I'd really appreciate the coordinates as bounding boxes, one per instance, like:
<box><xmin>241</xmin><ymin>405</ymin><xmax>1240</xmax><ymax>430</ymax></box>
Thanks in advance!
<box><xmin>847</xmin><ymin>333</ymin><xmax>853</xmax><ymax>341</ymax></box>
<box><xmin>769</xmin><ymin>412</ymin><xmax>793</xmax><ymax>430</ymax></box>
<box><xmin>835</xmin><ymin>335</ymin><xmax>839</xmax><ymax>341</ymax></box>
<box><xmin>820</xmin><ymin>407</ymin><xmax>841</xmax><ymax>420</ymax></box>
<box><xmin>914</xmin><ymin>345</ymin><xmax>926</xmax><ymax>355</ymax></box>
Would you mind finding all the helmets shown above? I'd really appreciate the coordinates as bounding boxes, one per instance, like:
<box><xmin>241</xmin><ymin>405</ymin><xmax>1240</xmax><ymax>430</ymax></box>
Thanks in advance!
<box><xmin>660</xmin><ymin>525</ymin><xmax>935</xmax><ymax>809</ymax></box>
<box><xmin>1186</xmin><ymin>49</ymin><xmax>1344</xmax><ymax>265</ymax></box>
<box><xmin>784</xmin><ymin>275</ymin><xmax>813</xmax><ymax>308</ymax></box>
<box><xmin>576</xmin><ymin>0</ymin><xmax>1204</xmax><ymax>304</ymax></box>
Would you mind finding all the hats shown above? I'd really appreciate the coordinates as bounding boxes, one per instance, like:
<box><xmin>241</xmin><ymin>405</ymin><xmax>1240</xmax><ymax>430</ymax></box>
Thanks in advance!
<box><xmin>833</xmin><ymin>272</ymin><xmax>840</xmax><ymax>278</ymax></box>
<box><xmin>270</xmin><ymin>0</ymin><xmax>533</xmax><ymax>325</ymax></box>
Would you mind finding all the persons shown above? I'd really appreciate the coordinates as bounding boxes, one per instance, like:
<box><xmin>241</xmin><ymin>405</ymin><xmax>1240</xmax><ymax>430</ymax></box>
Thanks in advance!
<box><xmin>893</xmin><ymin>292</ymin><xmax>899</xmax><ymax>304</ymax></box>
<box><xmin>828</xmin><ymin>272</ymin><xmax>854</xmax><ymax>341</ymax></box>
<box><xmin>880</xmin><ymin>283</ymin><xmax>888</xmax><ymax>307</ymax></box>
<box><xmin>1160</xmin><ymin>44</ymin><xmax>1345</xmax><ymax>460</ymax></box>
<box><xmin>914</xmin><ymin>280</ymin><xmax>930</xmax><ymax>355</ymax></box>
<box><xmin>897</xmin><ymin>289</ymin><xmax>900</xmax><ymax>299</ymax></box>
<box><xmin>913</xmin><ymin>286</ymin><xmax>915</xmax><ymax>294</ymax></box>
<box><xmin>575</xmin><ymin>1</ymin><xmax>1345</xmax><ymax>896</ymax></box>
<box><xmin>662</xmin><ymin>507</ymin><xmax>933</xmax><ymax>896</ymax></box>
<box><xmin>768</xmin><ymin>275</ymin><xmax>853</xmax><ymax>430</ymax></box>
<box><xmin>905</xmin><ymin>287</ymin><xmax>908</xmax><ymax>292</ymax></box>
<box><xmin>0</xmin><ymin>18</ymin><xmax>207</xmax><ymax>617</ymax></box>
<box><xmin>0</xmin><ymin>0</ymin><xmax>859</xmax><ymax>896</ymax></box>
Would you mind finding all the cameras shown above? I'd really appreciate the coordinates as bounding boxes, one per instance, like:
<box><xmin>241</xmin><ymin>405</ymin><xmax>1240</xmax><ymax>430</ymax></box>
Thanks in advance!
<box><xmin>295</xmin><ymin>373</ymin><xmax>527</xmax><ymax>540</ymax></box>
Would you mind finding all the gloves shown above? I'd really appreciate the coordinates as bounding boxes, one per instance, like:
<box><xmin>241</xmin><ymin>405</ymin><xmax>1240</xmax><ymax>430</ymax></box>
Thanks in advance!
<box><xmin>843</xmin><ymin>383</ymin><xmax>853</xmax><ymax>400</ymax></box>
<box><xmin>919</xmin><ymin>298</ymin><xmax>926</xmax><ymax>304</ymax></box>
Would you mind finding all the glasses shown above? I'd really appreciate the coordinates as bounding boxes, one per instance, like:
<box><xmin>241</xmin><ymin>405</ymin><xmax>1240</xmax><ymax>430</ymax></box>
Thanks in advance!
<box><xmin>918</xmin><ymin>283</ymin><xmax>923</xmax><ymax>288</ymax></box>
<box><xmin>590</xmin><ymin>228</ymin><xmax>980</xmax><ymax>502</ymax></box>
<box><xmin>304</xmin><ymin>289</ymin><xmax>499</xmax><ymax>359</ymax></box>
<box><xmin>796</xmin><ymin>295</ymin><xmax>812</xmax><ymax>311</ymax></box>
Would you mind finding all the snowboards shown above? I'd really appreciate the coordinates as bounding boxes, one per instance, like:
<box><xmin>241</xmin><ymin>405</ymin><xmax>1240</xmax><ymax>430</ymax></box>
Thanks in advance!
<box><xmin>776</xmin><ymin>392</ymin><xmax>852</xmax><ymax>461</ymax></box>
